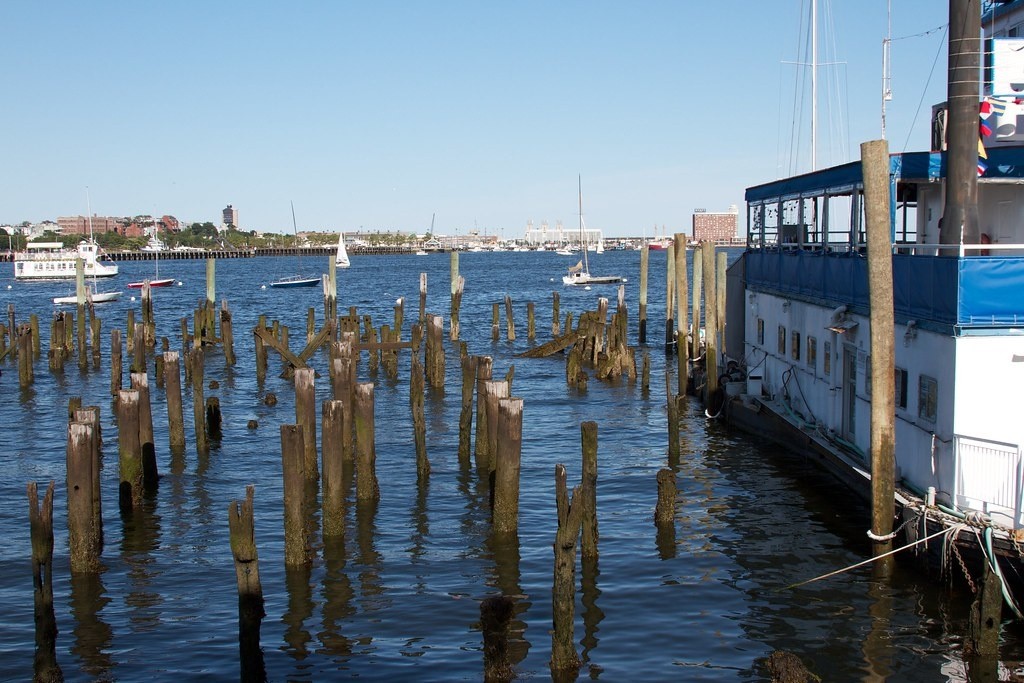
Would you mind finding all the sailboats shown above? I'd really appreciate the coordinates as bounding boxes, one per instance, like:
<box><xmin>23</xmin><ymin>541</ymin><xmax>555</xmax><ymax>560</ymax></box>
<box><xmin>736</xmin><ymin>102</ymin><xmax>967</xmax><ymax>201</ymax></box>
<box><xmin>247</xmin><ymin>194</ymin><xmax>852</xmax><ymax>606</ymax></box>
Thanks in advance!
<box><xmin>562</xmin><ymin>174</ymin><xmax>622</xmax><ymax>285</ymax></box>
<box><xmin>268</xmin><ymin>200</ymin><xmax>322</xmax><ymax>288</ymax></box>
<box><xmin>127</xmin><ymin>217</ymin><xmax>176</xmax><ymax>288</ymax></box>
<box><xmin>335</xmin><ymin>232</ymin><xmax>353</xmax><ymax>269</ymax></box>
<box><xmin>50</xmin><ymin>184</ymin><xmax>124</xmax><ymax>304</ymax></box>
<box><xmin>596</xmin><ymin>234</ymin><xmax>605</xmax><ymax>254</ymax></box>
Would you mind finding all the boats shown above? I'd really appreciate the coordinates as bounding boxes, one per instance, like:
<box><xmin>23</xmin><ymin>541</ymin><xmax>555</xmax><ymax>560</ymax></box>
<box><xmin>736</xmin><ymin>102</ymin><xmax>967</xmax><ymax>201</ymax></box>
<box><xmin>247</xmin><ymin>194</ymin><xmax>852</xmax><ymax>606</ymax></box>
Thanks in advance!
<box><xmin>742</xmin><ymin>0</ymin><xmax>1024</xmax><ymax>562</ymax></box>
<box><xmin>468</xmin><ymin>246</ymin><xmax>547</xmax><ymax>252</ymax></box>
<box><xmin>13</xmin><ymin>236</ymin><xmax>119</xmax><ymax>279</ymax></box>
<box><xmin>556</xmin><ymin>225</ymin><xmax>573</xmax><ymax>255</ymax></box>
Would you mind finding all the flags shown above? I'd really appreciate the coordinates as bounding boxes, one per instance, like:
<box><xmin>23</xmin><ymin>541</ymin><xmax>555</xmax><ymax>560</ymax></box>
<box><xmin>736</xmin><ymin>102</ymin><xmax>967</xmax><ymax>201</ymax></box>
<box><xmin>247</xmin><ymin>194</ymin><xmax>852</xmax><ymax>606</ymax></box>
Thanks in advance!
<box><xmin>977</xmin><ymin>97</ymin><xmax>1024</xmax><ymax>179</ymax></box>
